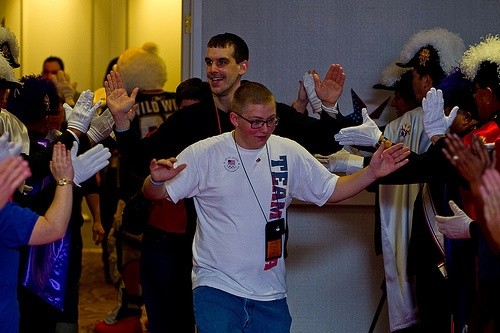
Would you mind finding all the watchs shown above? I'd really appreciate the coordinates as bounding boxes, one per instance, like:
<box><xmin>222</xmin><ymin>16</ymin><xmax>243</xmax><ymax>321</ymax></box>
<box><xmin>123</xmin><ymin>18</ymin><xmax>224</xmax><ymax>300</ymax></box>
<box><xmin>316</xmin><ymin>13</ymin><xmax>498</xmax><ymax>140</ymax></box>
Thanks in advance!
<box><xmin>56</xmin><ymin>179</ymin><xmax>73</xmax><ymax>185</ymax></box>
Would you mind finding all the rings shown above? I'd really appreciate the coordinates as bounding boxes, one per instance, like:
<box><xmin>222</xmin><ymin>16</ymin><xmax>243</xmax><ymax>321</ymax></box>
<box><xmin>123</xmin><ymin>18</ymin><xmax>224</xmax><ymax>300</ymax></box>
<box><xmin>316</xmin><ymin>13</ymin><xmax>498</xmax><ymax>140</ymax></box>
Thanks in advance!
<box><xmin>453</xmin><ymin>156</ymin><xmax>459</xmax><ymax>159</ymax></box>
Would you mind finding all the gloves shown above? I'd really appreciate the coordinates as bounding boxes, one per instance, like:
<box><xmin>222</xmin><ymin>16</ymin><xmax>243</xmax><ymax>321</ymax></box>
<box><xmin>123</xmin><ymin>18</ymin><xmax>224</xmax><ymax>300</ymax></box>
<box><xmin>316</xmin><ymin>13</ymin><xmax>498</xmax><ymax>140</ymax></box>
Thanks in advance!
<box><xmin>303</xmin><ymin>71</ymin><xmax>323</xmax><ymax>113</ymax></box>
<box><xmin>63</xmin><ymin>89</ymin><xmax>119</xmax><ymax>143</ymax></box>
<box><xmin>50</xmin><ymin>69</ymin><xmax>79</xmax><ymax>98</ymax></box>
<box><xmin>313</xmin><ymin>108</ymin><xmax>382</xmax><ymax>176</ymax></box>
<box><xmin>67</xmin><ymin>141</ymin><xmax>112</xmax><ymax>187</ymax></box>
<box><xmin>435</xmin><ymin>200</ymin><xmax>471</xmax><ymax>240</ymax></box>
<box><xmin>422</xmin><ymin>88</ymin><xmax>459</xmax><ymax>139</ymax></box>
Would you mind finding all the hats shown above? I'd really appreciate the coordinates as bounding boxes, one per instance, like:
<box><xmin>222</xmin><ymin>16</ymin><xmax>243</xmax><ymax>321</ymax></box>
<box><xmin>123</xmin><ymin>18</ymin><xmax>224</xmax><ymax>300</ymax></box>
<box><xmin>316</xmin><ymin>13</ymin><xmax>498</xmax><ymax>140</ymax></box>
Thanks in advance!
<box><xmin>372</xmin><ymin>43</ymin><xmax>499</xmax><ymax>110</ymax></box>
<box><xmin>114</xmin><ymin>41</ymin><xmax>168</xmax><ymax>95</ymax></box>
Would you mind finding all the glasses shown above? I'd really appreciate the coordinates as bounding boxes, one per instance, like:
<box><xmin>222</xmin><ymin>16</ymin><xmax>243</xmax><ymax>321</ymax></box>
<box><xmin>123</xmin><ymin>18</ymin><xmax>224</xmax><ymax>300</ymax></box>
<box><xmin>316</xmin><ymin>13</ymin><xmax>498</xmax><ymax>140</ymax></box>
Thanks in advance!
<box><xmin>235</xmin><ymin>113</ymin><xmax>278</xmax><ymax>129</ymax></box>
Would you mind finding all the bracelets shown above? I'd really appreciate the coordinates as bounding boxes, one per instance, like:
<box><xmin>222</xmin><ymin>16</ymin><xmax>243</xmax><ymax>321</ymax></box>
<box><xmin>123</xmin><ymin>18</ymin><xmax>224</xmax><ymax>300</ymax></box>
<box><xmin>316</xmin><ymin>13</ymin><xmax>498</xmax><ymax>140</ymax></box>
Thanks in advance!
<box><xmin>320</xmin><ymin>102</ymin><xmax>338</xmax><ymax>115</ymax></box>
<box><xmin>376</xmin><ymin>138</ymin><xmax>387</xmax><ymax>148</ymax></box>
<box><xmin>148</xmin><ymin>177</ymin><xmax>165</xmax><ymax>187</ymax></box>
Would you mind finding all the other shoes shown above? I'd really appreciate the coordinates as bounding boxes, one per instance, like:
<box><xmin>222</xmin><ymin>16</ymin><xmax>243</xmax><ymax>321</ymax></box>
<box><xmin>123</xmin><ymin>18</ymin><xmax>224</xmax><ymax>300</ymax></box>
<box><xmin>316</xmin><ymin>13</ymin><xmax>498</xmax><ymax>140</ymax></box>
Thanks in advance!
<box><xmin>102</xmin><ymin>304</ymin><xmax>141</xmax><ymax>325</ymax></box>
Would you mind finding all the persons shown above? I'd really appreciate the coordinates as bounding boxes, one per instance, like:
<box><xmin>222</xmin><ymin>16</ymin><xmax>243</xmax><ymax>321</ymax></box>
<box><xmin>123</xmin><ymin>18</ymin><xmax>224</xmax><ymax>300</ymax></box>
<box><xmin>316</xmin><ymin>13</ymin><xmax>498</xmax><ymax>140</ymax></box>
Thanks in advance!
<box><xmin>0</xmin><ymin>28</ymin><xmax>500</xmax><ymax>333</ymax></box>
